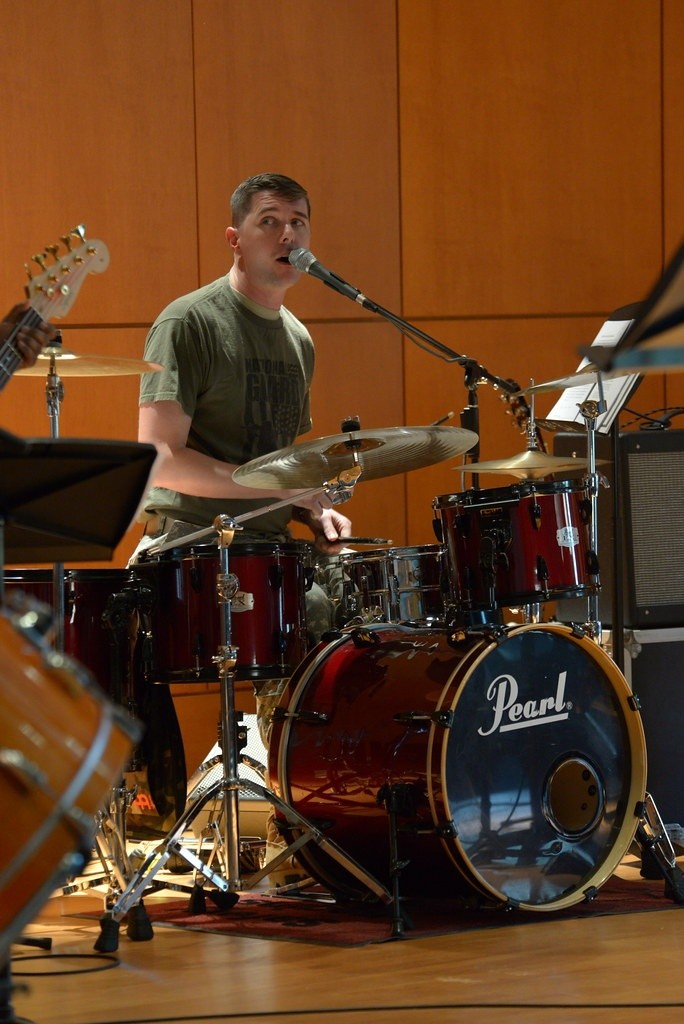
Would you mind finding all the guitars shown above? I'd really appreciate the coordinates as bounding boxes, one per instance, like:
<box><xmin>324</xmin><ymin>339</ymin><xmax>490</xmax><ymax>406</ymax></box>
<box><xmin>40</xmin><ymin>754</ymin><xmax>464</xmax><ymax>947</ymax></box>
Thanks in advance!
<box><xmin>0</xmin><ymin>224</ymin><xmax>112</xmax><ymax>392</ymax></box>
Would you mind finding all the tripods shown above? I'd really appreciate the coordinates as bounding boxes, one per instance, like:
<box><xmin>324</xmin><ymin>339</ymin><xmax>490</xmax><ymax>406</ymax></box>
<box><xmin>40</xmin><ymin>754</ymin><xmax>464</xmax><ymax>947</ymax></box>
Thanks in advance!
<box><xmin>92</xmin><ymin>466</ymin><xmax>410</xmax><ymax>955</ymax></box>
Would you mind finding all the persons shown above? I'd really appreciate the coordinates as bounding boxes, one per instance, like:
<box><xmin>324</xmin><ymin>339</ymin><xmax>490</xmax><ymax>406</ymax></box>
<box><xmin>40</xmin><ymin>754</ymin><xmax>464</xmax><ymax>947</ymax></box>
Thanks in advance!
<box><xmin>0</xmin><ymin>303</ymin><xmax>54</xmax><ymax>369</ymax></box>
<box><xmin>135</xmin><ymin>172</ymin><xmax>355</xmax><ymax>895</ymax></box>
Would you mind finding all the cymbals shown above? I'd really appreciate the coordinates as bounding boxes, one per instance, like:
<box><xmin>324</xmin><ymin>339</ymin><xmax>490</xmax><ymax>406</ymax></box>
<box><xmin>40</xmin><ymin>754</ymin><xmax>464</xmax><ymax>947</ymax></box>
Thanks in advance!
<box><xmin>520</xmin><ymin>363</ymin><xmax>684</xmax><ymax>390</ymax></box>
<box><xmin>7</xmin><ymin>349</ymin><xmax>165</xmax><ymax>378</ymax></box>
<box><xmin>231</xmin><ymin>425</ymin><xmax>479</xmax><ymax>490</ymax></box>
<box><xmin>452</xmin><ymin>450</ymin><xmax>607</xmax><ymax>489</ymax></box>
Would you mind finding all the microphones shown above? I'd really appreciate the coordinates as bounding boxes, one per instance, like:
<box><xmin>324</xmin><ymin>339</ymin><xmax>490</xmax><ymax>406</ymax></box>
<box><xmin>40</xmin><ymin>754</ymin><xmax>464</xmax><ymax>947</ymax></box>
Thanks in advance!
<box><xmin>287</xmin><ymin>248</ymin><xmax>378</xmax><ymax>312</ymax></box>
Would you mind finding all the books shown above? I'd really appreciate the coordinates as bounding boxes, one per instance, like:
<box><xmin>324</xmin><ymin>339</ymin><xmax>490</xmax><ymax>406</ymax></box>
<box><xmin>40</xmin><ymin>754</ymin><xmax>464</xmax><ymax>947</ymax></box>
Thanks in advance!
<box><xmin>543</xmin><ymin>319</ymin><xmax>641</xmax><ymax>434</ymax></box>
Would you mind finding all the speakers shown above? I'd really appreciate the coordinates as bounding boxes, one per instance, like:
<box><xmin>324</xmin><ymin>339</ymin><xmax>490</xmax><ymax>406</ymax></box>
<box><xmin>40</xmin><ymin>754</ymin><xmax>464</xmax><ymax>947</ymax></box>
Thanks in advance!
<box><xmin>552</xmin><ymin>429</ymin><xmax>684</xmax><ymax>628</ymax></box>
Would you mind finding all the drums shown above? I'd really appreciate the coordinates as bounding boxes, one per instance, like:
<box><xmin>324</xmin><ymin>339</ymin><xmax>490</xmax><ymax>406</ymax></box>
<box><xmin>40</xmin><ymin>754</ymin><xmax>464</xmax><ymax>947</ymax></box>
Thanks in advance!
<box><xmin>266</xmin><ymin>620</ymin><xmax>648</xmax><ymax>916</ymax></box>
<box><xmin>0</xmin><ymin>562</ymin><xmax>127</xmax><ymax>668</ymax></box>
<box><xmin>128</xmin><ymin>540</ymin><xmax>313</xmax><ymax>681</ymax></box>
<box><xmin>324</xmin><ymin>541</ymin><xmax>465</xmax><ymax>628</ymax></box>
<box><xmin>1</xmin><ymin>617</ymin><xmax>137</xmax><ymax>950</ymax></box>
<box><xmin>431</xmin><ymin>477</ymin><xmax>603</xmax><ymax>610</ymax></box>
